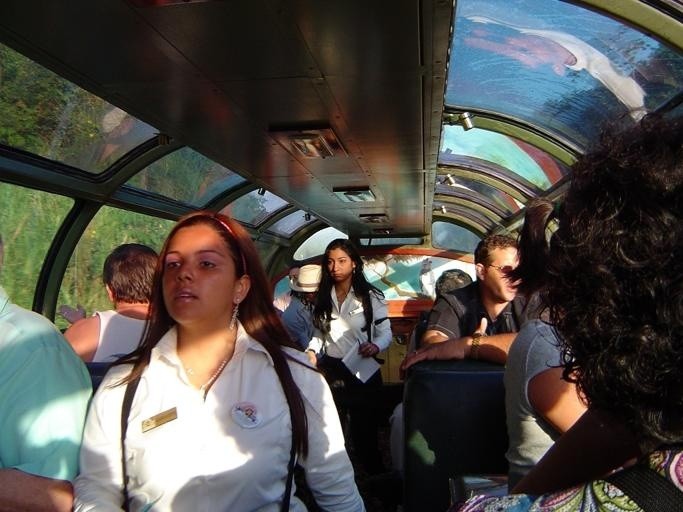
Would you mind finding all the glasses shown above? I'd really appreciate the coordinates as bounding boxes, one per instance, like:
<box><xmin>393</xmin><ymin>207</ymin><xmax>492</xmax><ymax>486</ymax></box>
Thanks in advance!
<box><xmin>489</xmin><ymin>263</ymin><xmax>512</xmax><ymax>276</ymax></box>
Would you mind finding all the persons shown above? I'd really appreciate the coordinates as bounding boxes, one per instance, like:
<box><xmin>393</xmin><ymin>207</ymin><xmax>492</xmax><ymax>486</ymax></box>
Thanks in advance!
<box><xmin>504</xmin><ymin>196</ymin><xmax>589</xmax><ymax>496</ymax></box>
<box><xmin>391</xmin><ymin>233</ymin><xmax>527</xmax><ymax>511</ymax></box>
<box><xmin>466</xmin><ymin>11</ymin><xmax>656</xmax><ymax>123</ymax></box>
<box><xmin>70</xmin><ymin>209</ymin><xmax>370</xmax><ymax>511</ymax></box>
<box><xmin>274</xmin><ymin>238</ymin><xmax>390</xmax><ymax>494</ymax></box>
<box><xmin>91</xmin><ymin>109</ymin><xmax>160</xmax><ymax>163</ymax></box>
<box><xmin>58</xmin><ymin>242</ymin><xmax>164</xmax><ymax>363</ymax></box>
<box><xmin>0</xmin><ymin>227</ymin><xmax>93</xmax><ymax>511</ymax></box>
<box><xmin>451</xmin><ymin>110</ymin><xmax>681</xmax><ymax>511</ymax></box>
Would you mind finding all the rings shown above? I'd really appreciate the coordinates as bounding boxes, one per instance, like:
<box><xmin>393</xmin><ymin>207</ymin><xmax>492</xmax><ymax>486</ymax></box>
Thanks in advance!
<box><xmin>64</xmin><ymin>312</ymin><xmax>69</xmax><ymax>315</ymax></box>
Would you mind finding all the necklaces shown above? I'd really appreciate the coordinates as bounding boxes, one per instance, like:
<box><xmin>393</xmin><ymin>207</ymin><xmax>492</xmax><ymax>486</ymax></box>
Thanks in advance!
<box><xmin>186</xmin><ymin>357</ymin><xmax>225</xmax><ymax>399</ymax></box>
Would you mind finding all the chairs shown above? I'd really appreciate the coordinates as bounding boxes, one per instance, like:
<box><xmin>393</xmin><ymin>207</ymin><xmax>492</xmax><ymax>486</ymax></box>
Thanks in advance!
<box><xmin>406</xmin><ymin>361</ymin><xmax>512</xmax><ymax>510</ymax></box>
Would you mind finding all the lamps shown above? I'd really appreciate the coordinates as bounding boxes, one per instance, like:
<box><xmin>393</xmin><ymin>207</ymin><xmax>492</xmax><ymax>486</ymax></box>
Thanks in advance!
<box><xmin>436</xmin><ymin>174</ymin><xmax>455</xmax><ymax>185</ymax></box>
<box><xmin>442</xmin><ymin>110</ymin><xmax>474</xmax><ymax>131</ymax></box>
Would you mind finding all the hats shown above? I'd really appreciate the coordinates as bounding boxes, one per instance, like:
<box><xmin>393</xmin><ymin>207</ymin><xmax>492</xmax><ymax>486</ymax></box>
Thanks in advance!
<box><xmin>289</xmin><ymin>265</ymin><xmax>322</xmax><ymax>293</ymax></box>
